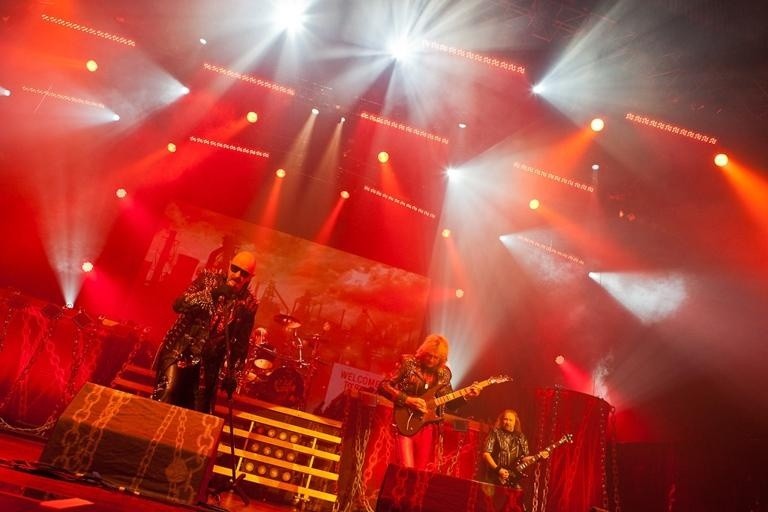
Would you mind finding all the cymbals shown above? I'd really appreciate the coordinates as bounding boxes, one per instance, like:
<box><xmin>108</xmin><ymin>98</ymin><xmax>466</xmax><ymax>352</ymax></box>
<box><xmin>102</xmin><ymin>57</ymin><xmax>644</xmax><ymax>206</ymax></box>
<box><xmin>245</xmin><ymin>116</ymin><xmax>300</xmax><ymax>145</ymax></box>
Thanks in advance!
<box><xmin>303</xmin><ymin>331</ymin><xmax>331</xmax><ymax>340</ymax></box>
<box><xmin>272</xmin><ymin>312</ymin><xmax>301</xmax><ymax>329</ymax></box>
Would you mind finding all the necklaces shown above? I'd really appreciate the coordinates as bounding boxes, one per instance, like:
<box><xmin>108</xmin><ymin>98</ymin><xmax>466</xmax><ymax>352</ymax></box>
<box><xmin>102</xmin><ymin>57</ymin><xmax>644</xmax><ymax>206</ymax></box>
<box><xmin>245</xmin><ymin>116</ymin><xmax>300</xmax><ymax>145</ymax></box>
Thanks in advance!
<box><xmin>424</xmin><ymin>382</ymin><xmax>429</xmax><ymax>390</ymax></box>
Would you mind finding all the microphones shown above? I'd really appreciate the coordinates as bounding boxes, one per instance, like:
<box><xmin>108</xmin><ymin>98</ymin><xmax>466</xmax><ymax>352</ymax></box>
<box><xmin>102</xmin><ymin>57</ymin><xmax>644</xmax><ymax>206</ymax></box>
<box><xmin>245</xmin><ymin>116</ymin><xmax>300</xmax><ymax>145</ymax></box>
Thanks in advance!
<box><xmin>218</xmin><ymin>280</ymin><xmax>235</xmax><ymax>302</ymax></box>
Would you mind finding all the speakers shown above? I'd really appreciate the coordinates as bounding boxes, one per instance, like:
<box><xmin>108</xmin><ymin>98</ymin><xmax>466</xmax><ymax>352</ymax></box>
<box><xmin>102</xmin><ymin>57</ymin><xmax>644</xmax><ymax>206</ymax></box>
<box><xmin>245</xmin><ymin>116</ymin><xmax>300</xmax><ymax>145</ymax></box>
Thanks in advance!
<box><xmin>375</xmin><ymin>463</ymin><xmax>524</xmax><ymax>512</ymax></box>
<box><xmin>39</xmin><ymin>381</ymin><xmax>226</xmax><ymax>506</ymax></box>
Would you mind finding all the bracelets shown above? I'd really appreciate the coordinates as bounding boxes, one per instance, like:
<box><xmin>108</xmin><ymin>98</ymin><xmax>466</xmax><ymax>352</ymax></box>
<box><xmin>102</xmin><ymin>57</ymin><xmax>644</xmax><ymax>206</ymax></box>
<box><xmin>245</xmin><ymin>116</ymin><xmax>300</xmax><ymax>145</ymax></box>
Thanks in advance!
<box><xmin>494</xmin><ymin>465</ymin><xmax>501</xmax><ymax>473</ymax></box>
<box><xmin>394</xmin><ymin>392</ymin><xmax>407</xmax><ymax>407</ymax></box>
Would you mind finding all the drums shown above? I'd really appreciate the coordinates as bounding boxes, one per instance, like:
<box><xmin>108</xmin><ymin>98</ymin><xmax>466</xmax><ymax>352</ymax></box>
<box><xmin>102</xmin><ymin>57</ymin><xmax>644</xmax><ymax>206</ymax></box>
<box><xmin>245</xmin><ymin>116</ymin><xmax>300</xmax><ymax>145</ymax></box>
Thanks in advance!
<box><xmin>254</xmin><ymin>343</ymin><xmax>277</xmax><ymax>369</ymax></box>
<box><xmin>261</xmin><ymin>366</ymin><xmax>305</xmax><ymax>407</ymax></box>
<box><xmin>277</xmin><ymin>353</ymin><xmax>296</xmax><ymax>368</ymax></box>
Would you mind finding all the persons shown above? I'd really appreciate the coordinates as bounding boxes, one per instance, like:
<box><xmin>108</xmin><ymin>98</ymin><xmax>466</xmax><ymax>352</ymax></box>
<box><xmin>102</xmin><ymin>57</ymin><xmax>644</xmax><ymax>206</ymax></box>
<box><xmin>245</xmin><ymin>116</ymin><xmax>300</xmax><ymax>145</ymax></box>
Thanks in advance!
<box><xmin>377</xmin><ymin>334</ymin><xmax>483</xmax><ymax>470</ymax></box>
<box><xmin>249</xmin><ymin>328</ymin><xmax>267</xmax><ymax>358</ymax></box>
<box><xmin>150</xmin><ymin>251</ymin><xmax>261</xmax><ymax>414</ymax></box>
<box><xmin>482</xmin><ymin>409</ymin><xmax>549</xmax><ymax>488</ymax></box>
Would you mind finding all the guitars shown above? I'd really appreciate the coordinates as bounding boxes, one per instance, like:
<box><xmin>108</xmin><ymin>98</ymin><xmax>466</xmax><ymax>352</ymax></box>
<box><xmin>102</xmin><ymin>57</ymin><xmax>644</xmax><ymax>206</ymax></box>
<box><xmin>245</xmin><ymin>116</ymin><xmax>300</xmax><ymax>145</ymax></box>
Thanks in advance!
<box><xmin>394</xmin><ymin>374</ymin><xmax>514</xmax><ymax>437</ymax></box>
<box><xmin>488</xmin><ymin>433</ymin><xmax>573</xmax><ymax>488</ymax></box>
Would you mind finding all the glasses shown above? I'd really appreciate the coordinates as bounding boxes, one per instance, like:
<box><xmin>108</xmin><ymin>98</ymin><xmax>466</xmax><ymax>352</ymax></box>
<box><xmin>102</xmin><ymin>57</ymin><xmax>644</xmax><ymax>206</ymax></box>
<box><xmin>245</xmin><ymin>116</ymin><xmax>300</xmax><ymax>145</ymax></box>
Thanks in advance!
<box><xmin>230</xmin><ymin>264</ymin><xmax>249</xmax><ymax>278</ymax></box>
